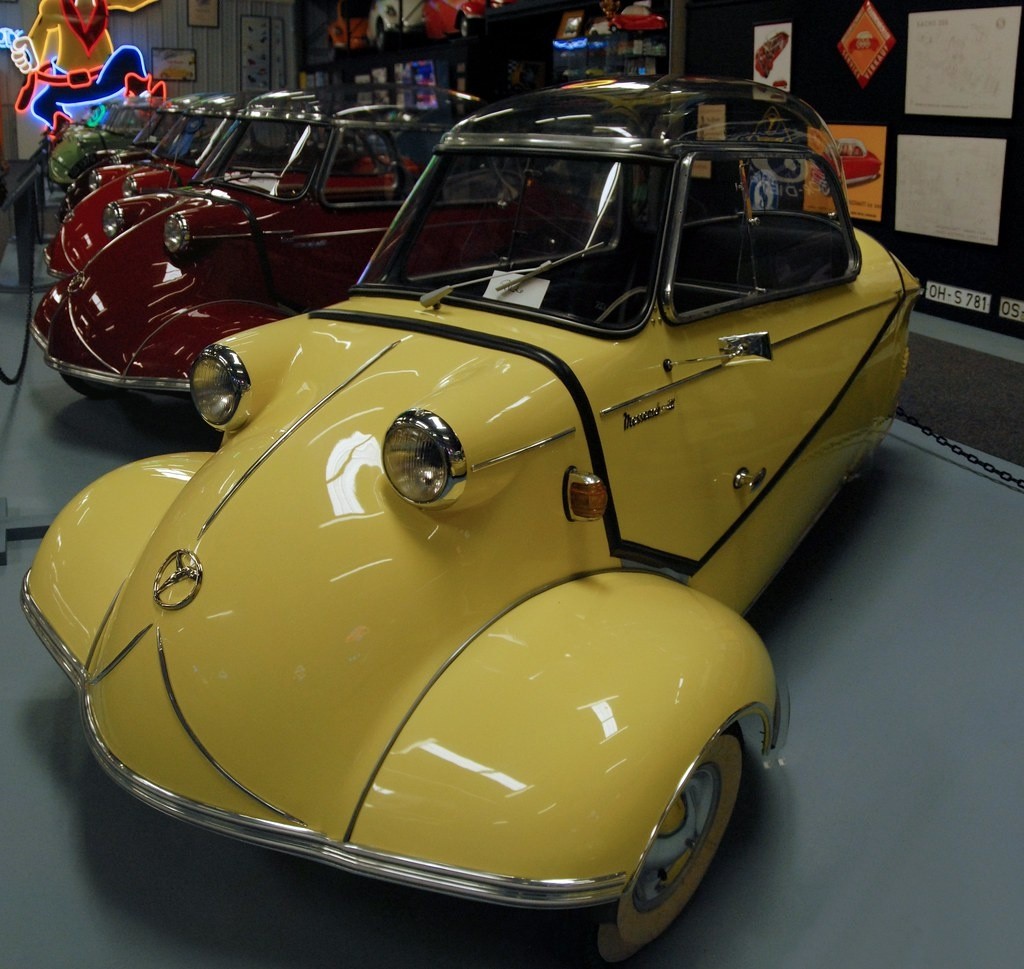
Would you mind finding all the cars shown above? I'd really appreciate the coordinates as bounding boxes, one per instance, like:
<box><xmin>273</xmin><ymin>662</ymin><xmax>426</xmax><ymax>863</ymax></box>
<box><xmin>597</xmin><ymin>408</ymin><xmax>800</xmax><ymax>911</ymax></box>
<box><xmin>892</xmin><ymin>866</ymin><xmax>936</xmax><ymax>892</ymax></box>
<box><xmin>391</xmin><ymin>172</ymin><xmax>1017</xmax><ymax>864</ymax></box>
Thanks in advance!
<box><xmin>16</xmin><ymin>71</ymin><xmax>930</xmax><ymax>969</ymax></box>
<box><xmin>812</xmin><ymin>137</ymin><xmax>883</xmax><ymax>197</ymax></box>
<box><xmin>28</xmin><ymin>74</ymin><xmax>647</xmax><ymax>402</ymax></box>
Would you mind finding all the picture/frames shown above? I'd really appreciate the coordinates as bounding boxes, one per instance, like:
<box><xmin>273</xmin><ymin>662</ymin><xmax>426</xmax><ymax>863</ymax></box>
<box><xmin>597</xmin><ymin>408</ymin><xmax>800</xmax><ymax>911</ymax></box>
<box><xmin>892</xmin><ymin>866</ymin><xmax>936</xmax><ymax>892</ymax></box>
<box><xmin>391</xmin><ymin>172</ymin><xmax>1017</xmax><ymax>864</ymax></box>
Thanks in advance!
<box><xmin>238</xmin><ymin>15</ymin><xmax>287</xmax><ymax>92</ymax></box>
<box><xmin>186</xmin><ymin>0</ymin><xmax>219</xmax><ymax>29</ymax></box>
<box><xmin>151</xmin><ymin>47</ymin><xmax>196</xmax><ymax>82</ymax></box>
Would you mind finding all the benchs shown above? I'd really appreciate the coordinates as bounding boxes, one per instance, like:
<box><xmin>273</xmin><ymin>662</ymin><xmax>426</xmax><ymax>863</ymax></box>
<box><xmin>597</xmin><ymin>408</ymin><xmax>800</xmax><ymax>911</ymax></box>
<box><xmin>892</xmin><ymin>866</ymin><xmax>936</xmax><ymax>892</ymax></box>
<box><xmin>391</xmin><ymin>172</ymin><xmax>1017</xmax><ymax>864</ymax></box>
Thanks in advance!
<box><xmin>679</xmin><ymin>223</ymin><xmax>848</xmax><ymax>291</ymax></box>
